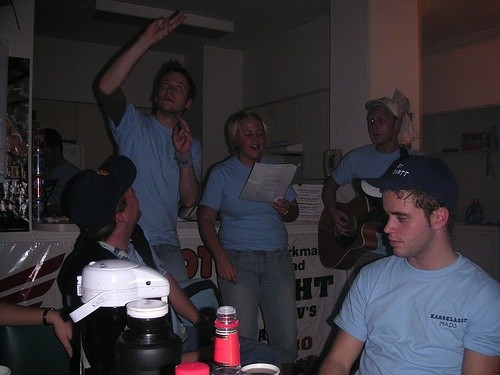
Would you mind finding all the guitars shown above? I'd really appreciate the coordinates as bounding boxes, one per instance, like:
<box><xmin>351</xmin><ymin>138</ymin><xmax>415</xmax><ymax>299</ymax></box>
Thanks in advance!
<box><xmin>317</xmin><ymin>196</ymin><xmax>390</xmax><ymax>271</ymax></box>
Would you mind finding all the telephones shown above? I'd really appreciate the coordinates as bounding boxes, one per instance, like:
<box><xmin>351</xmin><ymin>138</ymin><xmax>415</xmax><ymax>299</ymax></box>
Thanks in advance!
<box><xmin>323</xmin><ymin>148</ymin><xmax>342</xmax><ymax>178</ymax></box>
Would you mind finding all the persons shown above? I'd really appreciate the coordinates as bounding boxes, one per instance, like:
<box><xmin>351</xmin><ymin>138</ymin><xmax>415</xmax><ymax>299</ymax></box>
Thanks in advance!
<box><xmin>57</xmin><ymin>154</ymin><xmax>282</xmax><ymax>375</ymax></box>
<box><xmin>0</xmin><ymin>299</ymin><xmax>73</xmax><ymax>375</ymax></box>
<box><xmin>317</xmin><ymin>153</ymin><xmax>500</xmax><ymax>375</ymax></box>
<box><xmin>99</xmin><ymin>8</ymin><xmax>203</xmax><ymax>361</ymax></box>
<box><xmin>197</xmin><ymin>110</ymin><xmax>300</xmax><ymax>375</ymax></box>
<box><xmin>39</xmin><ymin>127</ymin><xmax>84</xmax><ymax>216</ymax></box>
<box><xmin>321</xmin><ymin>96</ymin><xmax>426</xmax><ymax>275</ymax></box>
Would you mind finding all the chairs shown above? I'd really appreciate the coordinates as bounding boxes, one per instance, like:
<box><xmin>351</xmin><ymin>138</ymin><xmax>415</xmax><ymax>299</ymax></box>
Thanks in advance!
<box><xmin>0</xmin><ymin>305</ymin><xmax>76</xmax><ymax>375</ymax></box>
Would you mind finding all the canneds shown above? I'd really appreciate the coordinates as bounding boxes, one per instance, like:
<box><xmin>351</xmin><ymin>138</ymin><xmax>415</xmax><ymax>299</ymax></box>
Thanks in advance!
<box><xmin>32</xmin><ymin>127</ymin><xmax>44</xmax><ymax>221</ymax></box>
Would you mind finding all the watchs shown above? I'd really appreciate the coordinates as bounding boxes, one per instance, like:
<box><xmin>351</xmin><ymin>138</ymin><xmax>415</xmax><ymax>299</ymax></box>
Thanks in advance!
<box><xmin>42</xmin><ymin>306</ymin><xmax>56</xmax><ymax>326</ymax></box>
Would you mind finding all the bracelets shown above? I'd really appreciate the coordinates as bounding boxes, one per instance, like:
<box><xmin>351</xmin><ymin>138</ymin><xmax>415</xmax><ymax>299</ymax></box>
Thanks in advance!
<box><xmin>174</xmin><ymin>153</ymin><xmax>192</xmax><ymax>166</ymax></box>
<box><xmin>285</xmin><ymin>209</ymin><xmax>292</xmax><ymax>218</ymax></box>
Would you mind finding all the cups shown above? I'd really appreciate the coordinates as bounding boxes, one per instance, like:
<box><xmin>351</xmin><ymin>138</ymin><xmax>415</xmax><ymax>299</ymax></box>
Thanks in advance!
<box><xmin>241</xmin><ymin>363</ymin><xmax>281</xmax><ymax>375</ymax></box>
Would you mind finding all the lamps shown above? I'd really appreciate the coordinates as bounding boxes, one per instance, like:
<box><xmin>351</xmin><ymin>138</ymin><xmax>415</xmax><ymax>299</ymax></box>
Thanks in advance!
<box><xmin>94</xmin><ymin>0</ymin><xmax>235</xmax><ymax>33</ymax></box>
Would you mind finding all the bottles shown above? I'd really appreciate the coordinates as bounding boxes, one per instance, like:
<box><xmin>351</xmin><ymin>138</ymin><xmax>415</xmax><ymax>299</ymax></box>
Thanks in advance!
<box><xmin>210</xmin><ymin>306</ymin><xmax>244</xmax><ymax>375</ymax></box>
<box><xmin>175</xmin><ymin>361</ymin><xmax>209</xmax><ymax>375</ymax></box>
<box><xmin>111</xmin><ymin>296</ymin><xmax>182</xmax><ymax>375</ymax></box>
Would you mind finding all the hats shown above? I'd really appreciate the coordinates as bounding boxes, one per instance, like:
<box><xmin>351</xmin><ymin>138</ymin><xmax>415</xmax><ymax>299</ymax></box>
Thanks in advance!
<box><xmin>61</xmin><ymin>154</ymin><xmax>137</xmax><ymax>230</ymax></box>
<box><xmin>365</xmin><ymin>97</ymin><xmax>403</xmax><ymax>120</ymax></box>
<box><xmin>360</xmin><ymin>155</ymin><xmax>459</xmax><ymax>208</ymax></box>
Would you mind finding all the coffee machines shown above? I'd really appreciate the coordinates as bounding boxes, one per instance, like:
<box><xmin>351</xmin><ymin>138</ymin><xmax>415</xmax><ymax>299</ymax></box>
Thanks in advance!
<box><xmin>67</xmin><ymin>259</ymin><xmax>183</xmax><ymax>375</ymax></box>
